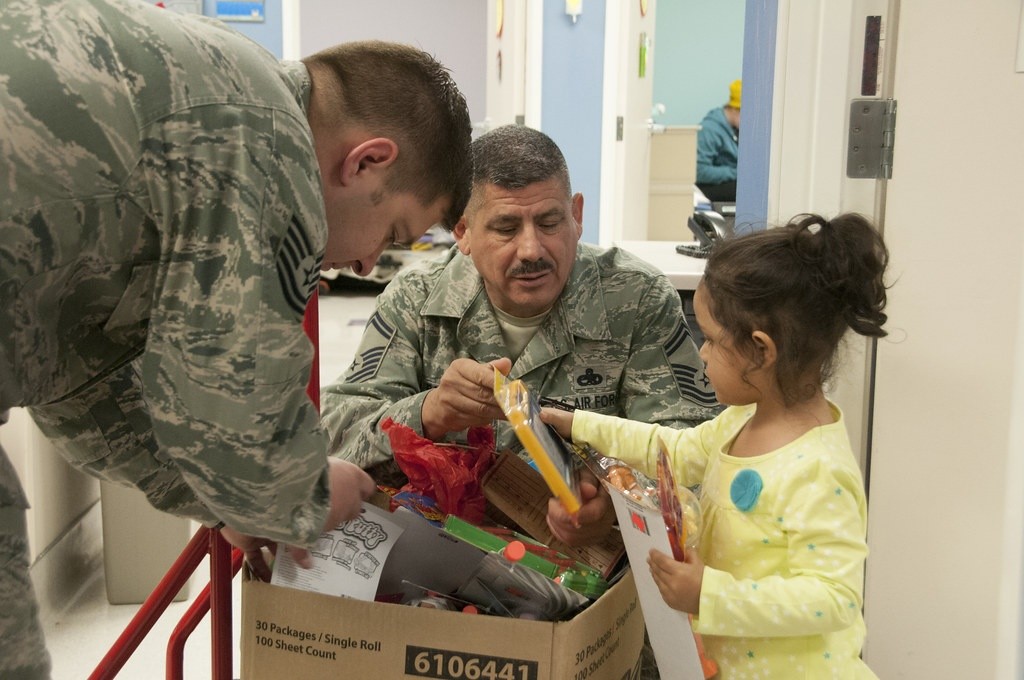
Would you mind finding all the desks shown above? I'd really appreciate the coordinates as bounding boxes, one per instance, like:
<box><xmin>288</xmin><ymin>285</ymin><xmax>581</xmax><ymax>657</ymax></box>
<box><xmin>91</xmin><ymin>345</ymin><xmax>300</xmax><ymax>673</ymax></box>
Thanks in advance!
<box><xmin>619</xmin><ymin>240</ymin><xmax>709</xmax><ymax>313</ymax></box>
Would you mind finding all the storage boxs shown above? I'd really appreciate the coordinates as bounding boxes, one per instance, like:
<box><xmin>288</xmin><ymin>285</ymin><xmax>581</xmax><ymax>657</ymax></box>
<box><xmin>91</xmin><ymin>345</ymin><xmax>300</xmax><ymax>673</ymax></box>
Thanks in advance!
<box><xmin>242</xmin><ymin>538</ymin><xmax>643</xmax><ymax>680</ymax></box>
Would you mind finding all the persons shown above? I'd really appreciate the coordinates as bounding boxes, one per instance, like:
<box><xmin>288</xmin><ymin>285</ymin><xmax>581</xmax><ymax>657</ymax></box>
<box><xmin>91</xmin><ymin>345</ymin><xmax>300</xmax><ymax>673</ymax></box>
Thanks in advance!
<box><xmin>320</xmin><ymin>124</ymin><xmax>720</xmax><ymax>545</ymax></box>
<box><xmin>540</xmin><ymin>211</ymin><xmax>908</xmax><ymax>680</ymax></box>
<box><xmin>0</xmin><ymin>0</ymin><xmax>474</xmax><ymax>680</ymax></box>
<box><xmin>695</xmin><ymin>79</ymin><xmax>744</xmax><ymax>203</ymax></box>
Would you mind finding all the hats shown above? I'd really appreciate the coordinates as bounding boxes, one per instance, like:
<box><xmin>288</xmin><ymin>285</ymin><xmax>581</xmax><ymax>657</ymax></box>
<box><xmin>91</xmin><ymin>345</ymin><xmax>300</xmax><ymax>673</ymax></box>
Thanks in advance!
<box><xmin>728</xmin><ymin>80</ymin><xmax>742</xmax><ymax>108</ymax></box>
